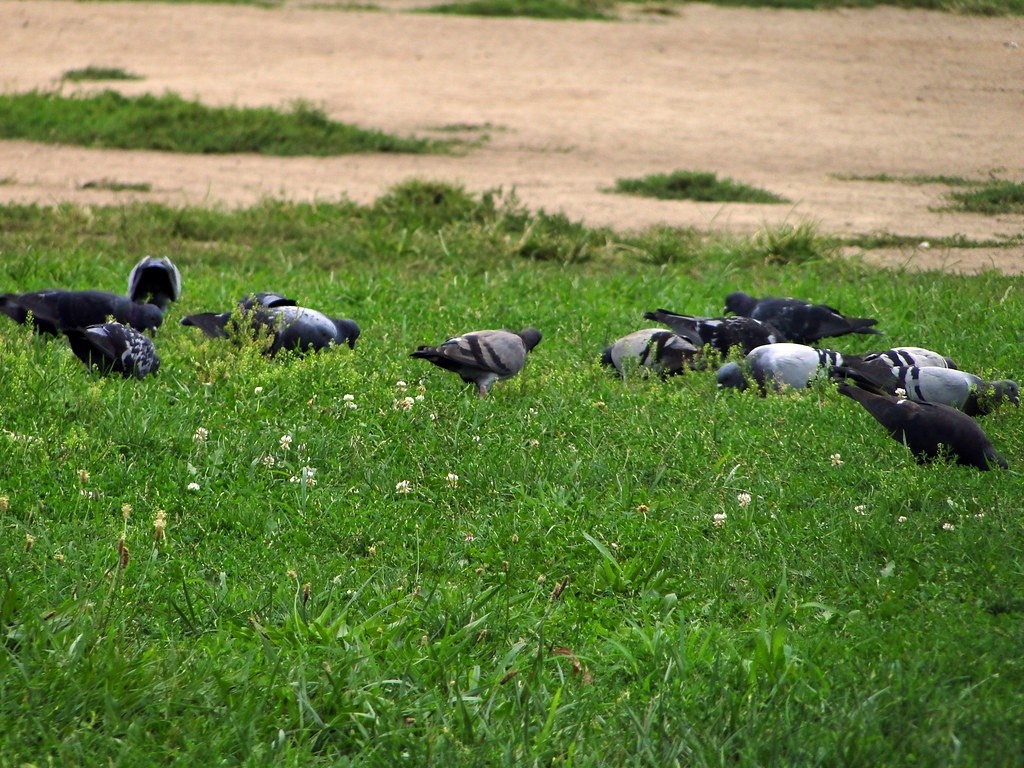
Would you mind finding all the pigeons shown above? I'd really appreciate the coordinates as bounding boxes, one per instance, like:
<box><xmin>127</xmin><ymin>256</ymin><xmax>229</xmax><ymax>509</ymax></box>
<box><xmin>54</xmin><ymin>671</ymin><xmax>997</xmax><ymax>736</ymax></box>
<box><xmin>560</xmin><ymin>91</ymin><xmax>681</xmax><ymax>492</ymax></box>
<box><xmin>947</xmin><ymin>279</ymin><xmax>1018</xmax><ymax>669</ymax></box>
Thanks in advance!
<box><xmin>0</xmin><ymin>253</ymin><xmax>1021</xmax><ymax>475</ymax></box>
